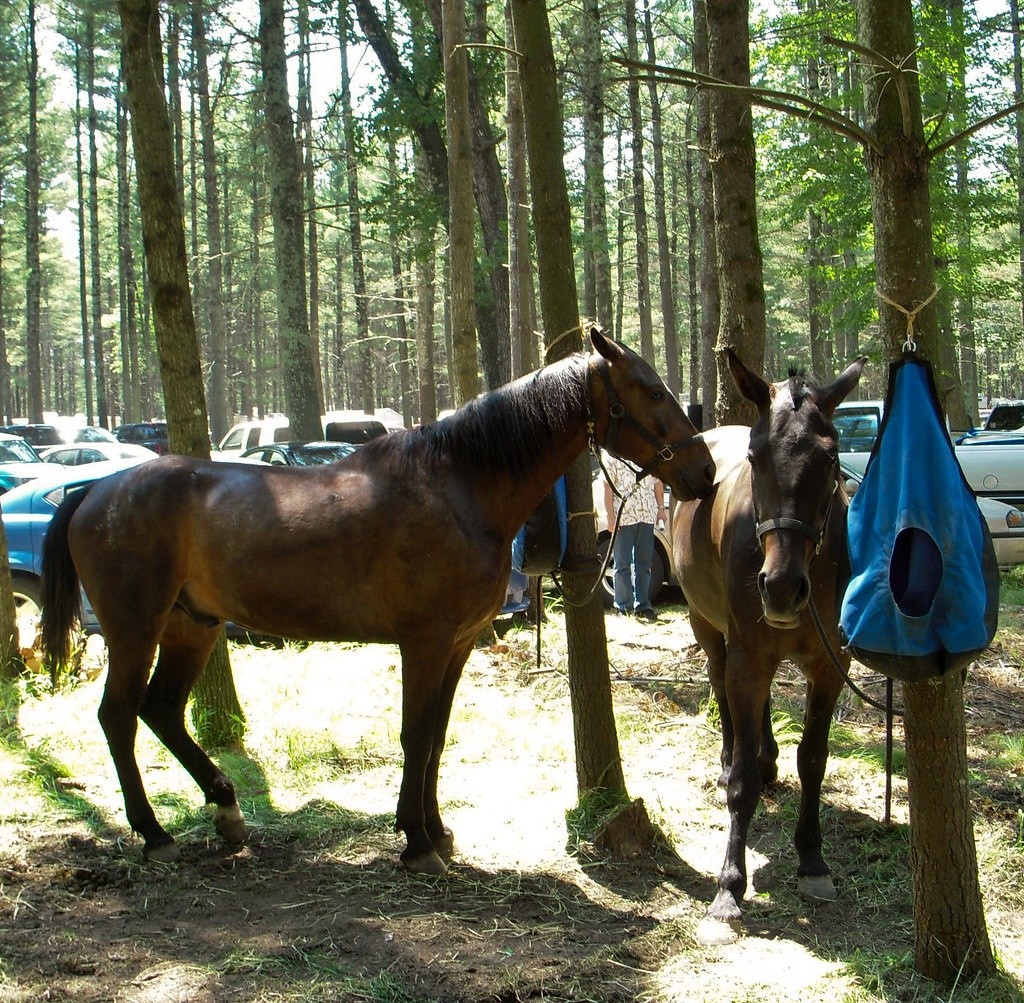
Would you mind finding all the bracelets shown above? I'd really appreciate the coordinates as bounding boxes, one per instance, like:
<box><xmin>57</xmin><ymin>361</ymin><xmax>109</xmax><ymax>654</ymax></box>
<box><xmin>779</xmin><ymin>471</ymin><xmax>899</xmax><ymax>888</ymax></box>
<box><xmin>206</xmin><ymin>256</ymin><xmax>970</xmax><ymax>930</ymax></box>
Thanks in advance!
<box><xmin>655</xmin><ymin>505</ymin><xmax>666</xmax><ymax>509</ymax></box>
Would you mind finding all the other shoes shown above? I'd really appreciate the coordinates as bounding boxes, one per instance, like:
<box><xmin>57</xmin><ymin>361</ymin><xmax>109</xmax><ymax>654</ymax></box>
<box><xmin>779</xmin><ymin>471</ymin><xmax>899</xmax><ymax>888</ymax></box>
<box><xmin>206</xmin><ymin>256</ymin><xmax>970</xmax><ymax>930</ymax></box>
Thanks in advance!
<box><xmin>617</xmin><ymin>609</ymin><xmax>630</xmax><ymax>616</ymax></box>
<box><xmin>635</xmin><ymin>609</ymin><xmax>657</xmax><ymax>621</ymax></box>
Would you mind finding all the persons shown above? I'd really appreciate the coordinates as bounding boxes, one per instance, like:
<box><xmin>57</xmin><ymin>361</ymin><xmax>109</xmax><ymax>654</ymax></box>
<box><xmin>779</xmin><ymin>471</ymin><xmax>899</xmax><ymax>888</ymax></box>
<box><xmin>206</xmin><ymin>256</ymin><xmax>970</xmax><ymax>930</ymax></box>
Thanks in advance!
<box><xmin>601</xmin><ymin>448</ymin><xmax>668</xmax><ymax>621</ymax></box>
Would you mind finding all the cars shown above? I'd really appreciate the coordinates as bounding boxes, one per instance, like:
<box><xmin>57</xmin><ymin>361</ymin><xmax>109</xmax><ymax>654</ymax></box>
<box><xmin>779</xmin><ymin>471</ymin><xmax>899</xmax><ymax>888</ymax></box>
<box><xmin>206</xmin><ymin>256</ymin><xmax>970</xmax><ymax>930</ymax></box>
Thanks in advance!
<box><xmin>1</xmin><ymin>398</ymin><xmax>1024</xmax><ymax>622</ymax></box>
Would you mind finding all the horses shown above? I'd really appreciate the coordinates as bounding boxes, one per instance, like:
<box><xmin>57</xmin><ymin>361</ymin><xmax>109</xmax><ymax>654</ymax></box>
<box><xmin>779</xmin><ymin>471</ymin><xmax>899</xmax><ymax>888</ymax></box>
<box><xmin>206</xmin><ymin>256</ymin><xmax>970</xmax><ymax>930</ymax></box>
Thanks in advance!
<box><xmin>669</xmin><ymin>343</ymin><xmax>871</xmax><ymax>944</ymax></box>
<box><xmin>33</xmin><ymin>326</ymin><xmax>719</xmax><ymax>873</ymax></box>
